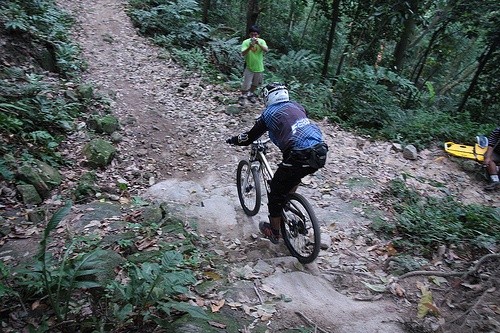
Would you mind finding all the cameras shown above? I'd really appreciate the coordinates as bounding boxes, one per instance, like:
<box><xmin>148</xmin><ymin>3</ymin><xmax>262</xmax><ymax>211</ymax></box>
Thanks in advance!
<box><xmin>250</xmin><ymin>37</ymin><xmax>257</xmax><ymax>43</ymax></box>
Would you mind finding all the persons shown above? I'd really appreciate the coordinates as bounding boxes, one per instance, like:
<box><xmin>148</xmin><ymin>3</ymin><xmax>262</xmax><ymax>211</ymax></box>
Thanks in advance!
<box><xmin>238</xmin><ymin>25</ymin><xmax>269</xmax><ymax>106</ymax></box>
<box><xmin>230</xmin><ymin>82</ymin><xmax>329</xmax><ymax>245</ymax></box>
<box><xmin>484</xmin><ymin>128</ymin><xmax>500</xmax><ymax>191</ymax></box>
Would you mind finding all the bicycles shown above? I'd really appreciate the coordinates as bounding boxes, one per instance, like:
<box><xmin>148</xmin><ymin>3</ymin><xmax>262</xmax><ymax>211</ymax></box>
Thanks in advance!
<box><xmin>225</xmin><ymin>136</ymin><xmax>320</xmax><ymax>263</ymax></box>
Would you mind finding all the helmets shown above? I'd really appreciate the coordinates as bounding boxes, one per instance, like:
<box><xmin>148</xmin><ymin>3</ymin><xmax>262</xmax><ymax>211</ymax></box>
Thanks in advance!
<box><xmin>260</xmin><ymin>80</ymin><xmax>289</xmax><ymax>105</ymax></box>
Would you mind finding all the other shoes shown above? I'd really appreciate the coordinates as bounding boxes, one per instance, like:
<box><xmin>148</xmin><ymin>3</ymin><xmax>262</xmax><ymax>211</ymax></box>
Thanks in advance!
<box><xmin>485</xmin><ymin>181</ymin><xmax>500</xmax><ymax>190</ymax></box>
<box><xmin>238</xmin><ymin>96</ymin><xmax>245</xmax><ymax>105</ymax></box>
<box><xmin>247</xmin><ymin>94</ymin><xmax>256</xmax><ymax>103</ymax></box>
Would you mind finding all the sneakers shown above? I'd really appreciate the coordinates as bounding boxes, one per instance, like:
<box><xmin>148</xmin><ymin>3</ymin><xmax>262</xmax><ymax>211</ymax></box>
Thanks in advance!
<box><xmin>259</xmin><ymin>220</ymin><xmax>280</xmax><ymax>244</ymax></box>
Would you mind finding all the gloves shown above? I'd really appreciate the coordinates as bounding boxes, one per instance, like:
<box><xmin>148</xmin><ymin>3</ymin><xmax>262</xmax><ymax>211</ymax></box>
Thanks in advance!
<box><xmin>230</xmin><ymin>135</ymin><xmax>238</xmax><ymax>144</ymax></box>
